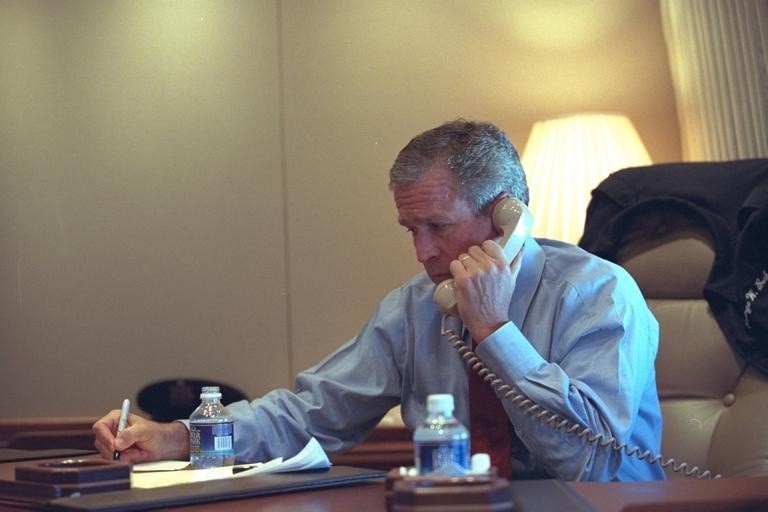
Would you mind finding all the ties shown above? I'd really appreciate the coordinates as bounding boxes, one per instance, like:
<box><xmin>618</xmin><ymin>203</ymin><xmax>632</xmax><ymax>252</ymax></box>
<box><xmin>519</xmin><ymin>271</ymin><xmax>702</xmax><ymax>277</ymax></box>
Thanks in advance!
<box><xmin>468</xmin><ymin>337</ymin><xmax>513</xmax><ymax>482</ymax></box>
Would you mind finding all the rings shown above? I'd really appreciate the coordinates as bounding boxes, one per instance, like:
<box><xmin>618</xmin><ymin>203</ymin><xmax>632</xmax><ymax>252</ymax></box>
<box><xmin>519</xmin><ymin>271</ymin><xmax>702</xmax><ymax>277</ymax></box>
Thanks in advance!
<box><xmin>461</xmin><ymin>256</ymin><xmax>470</xmax><ymax>264</ymax></box>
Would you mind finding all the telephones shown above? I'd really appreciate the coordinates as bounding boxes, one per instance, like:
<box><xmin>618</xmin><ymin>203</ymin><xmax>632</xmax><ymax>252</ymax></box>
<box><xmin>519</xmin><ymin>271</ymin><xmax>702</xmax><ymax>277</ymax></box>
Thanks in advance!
<box><xmin>431</xmin><ymin>196</ymin><xmax>534</xmax><ymax>317</ymax></box>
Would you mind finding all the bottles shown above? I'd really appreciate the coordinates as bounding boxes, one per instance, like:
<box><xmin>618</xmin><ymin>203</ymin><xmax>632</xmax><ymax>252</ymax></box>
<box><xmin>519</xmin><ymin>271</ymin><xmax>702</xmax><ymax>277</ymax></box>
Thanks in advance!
<box><xmin>189</xmin><ymin>384</ymin><xmax>235</xmax><ymax>469</ymax></box>
<box><xmin>412</xmin><ymin>393</ymin><xmax>473</xmax><ymax>478</ymax></box>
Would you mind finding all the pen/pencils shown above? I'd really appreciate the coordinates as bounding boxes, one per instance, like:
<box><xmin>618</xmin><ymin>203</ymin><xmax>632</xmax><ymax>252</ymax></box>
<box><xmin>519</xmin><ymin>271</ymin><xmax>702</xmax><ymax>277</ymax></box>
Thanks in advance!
<box><xmin>111</xmin><ymin>398</ymin><xmax>130</xmax><ymax>462</ymax></box>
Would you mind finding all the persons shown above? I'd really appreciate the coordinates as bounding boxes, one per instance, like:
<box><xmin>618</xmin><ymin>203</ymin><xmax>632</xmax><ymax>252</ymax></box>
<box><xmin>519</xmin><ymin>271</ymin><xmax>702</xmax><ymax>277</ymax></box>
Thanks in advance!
<box><xmin>93</xmin><ymin>119</ymin><xmax>668</xmax><ymax>481</ymax></box>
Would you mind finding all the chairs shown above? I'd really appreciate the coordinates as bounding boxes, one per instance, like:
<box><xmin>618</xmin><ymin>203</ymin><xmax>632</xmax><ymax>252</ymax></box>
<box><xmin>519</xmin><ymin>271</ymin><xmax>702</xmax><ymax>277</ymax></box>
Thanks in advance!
<box><xmin>586</xmin><ymin>157</ymin><xmax>766</xmax><ymax>477</ymax></box>
